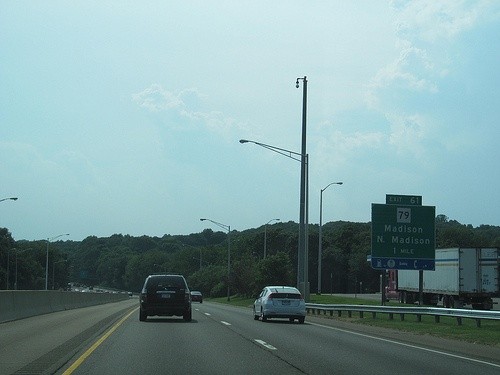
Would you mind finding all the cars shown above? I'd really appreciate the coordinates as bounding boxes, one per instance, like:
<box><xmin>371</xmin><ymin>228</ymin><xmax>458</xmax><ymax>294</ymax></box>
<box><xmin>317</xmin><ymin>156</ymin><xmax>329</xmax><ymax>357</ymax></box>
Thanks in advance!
<box><xmin>191</xmin><ymin>291</ymin><xmax>203</xmax><ymax>303</ymax></box>
<box><xmin>253</xmin><ymin>286</ymin><xmax>306</xmax><ymax>323</ymax></box>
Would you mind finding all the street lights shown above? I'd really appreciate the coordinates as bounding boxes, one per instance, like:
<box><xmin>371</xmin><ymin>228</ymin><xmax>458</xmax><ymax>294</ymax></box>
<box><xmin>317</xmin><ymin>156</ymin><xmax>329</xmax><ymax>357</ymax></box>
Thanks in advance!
<box><xmin>199</xmin><ymin>218</ymin><xmax>231</xmax><ymax>302</ymax></box>
<box><xmin>317</xmin><ymin>181</ymin><xmax>343</xmax><ymax>295</ymax></box>
<box><xmin>45</xmin><ymin>234</ymin><xmax>69</xmax><ymax>291</ymax></box>
<box><xmin>6</xmin><ymin>248</ymin><xmax>16</xmax><ymax>290</ymax></box>
<box><xmin>239</xmin><ymin>139</ymin><xmax>309</xmax><ymax>302</ymax></box>
<box><xmin>264</xmin><ymin>218</ymin><xmax>280</xmax><ymax>258</ymax></box>
<box><xmin>295</xmin><ymin>75</ymin><xmax>308</xmax><ymax>293</ymax></box>
<box><xmin>52</xmin><ymin>260</ymin><xmax>67</xmax><ymax>290</ymax></box>
<box><xmin>15</xmin><ymin>248</ymin><xmax>33</xmax><ymax>290</ymax></box>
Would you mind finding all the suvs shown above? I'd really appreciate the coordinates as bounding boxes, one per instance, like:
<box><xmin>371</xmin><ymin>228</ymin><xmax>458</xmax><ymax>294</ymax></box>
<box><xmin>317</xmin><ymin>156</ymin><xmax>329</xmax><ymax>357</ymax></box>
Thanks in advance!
<box><xmin>139</xmin><ymin>275</ymin><xmax>192</xmax><ymax>322</ymax></box>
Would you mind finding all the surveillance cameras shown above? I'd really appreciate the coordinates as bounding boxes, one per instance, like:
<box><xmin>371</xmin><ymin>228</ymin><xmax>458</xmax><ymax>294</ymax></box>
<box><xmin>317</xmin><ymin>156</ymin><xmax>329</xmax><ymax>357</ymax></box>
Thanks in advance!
<box><xmin>296</xmin><ymin>85</ymin><xmax>299</xmax><ymax>88</ymax></box>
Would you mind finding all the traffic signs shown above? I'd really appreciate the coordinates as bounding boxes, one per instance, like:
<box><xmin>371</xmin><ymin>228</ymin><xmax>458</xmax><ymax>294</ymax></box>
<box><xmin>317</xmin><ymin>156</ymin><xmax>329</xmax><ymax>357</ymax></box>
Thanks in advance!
<box><xmin>371</xmin><ymin>203</ymin><xmax>436</xmax><ymax>272</ymax></box>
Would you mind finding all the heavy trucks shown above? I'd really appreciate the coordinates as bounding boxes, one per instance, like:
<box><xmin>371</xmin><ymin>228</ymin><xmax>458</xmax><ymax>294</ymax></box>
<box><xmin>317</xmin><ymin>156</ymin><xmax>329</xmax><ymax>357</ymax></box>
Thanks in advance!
<box><xmin>383</xmin><ymin>247</ymin><xmax>500</xmax><ymax>310</ymax></box>
